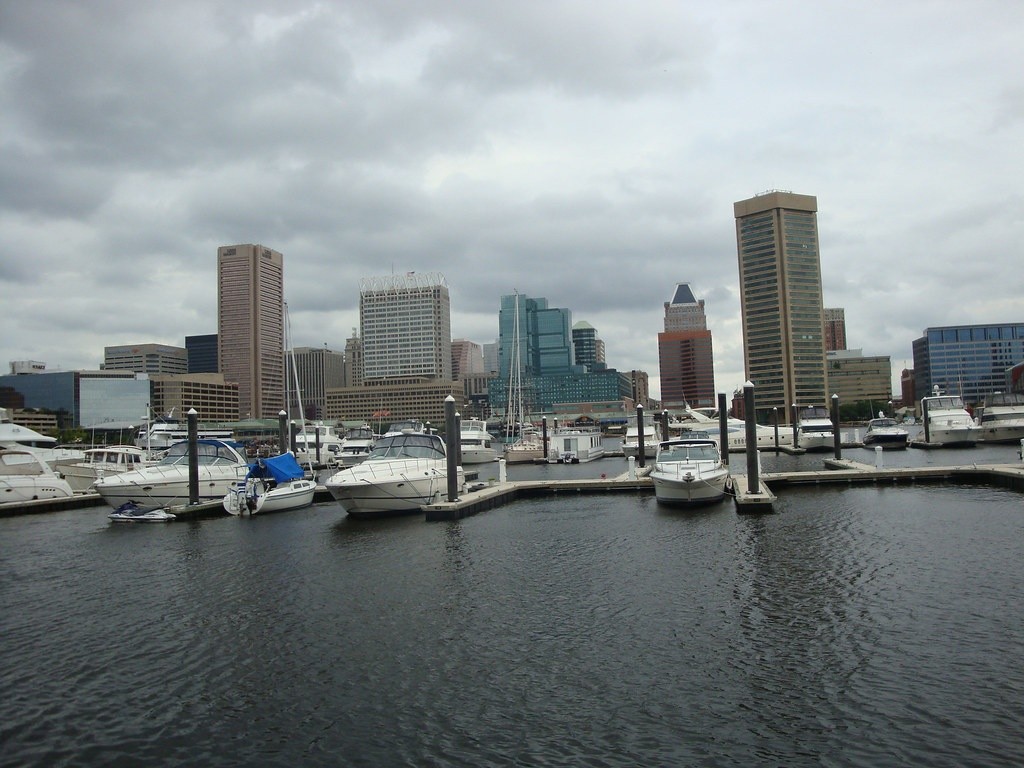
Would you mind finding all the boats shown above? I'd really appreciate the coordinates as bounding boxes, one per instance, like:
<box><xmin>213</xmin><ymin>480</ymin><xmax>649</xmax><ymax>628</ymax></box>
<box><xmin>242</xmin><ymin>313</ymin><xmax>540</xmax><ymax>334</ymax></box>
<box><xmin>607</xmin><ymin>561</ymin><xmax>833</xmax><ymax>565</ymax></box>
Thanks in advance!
<box><xmin>0</xmin><ymin>405</ymin><xmax>165</xmax><ymax>509</ymax></box>
<box><xmin>323</xmin><ymin>420</ymin><xmax>469</xmax><ymax>514</ymax></box>
<box><xmin>384</xmin><ymin>420</ymin><xmax>428</xmax><ymax>436</ymax></box>
<box><xmin>973</xmin><ymin>391</ymin><xmax>1024</xmax><ymax>443</ymax></box>
<box><xmin>671</xmin><ymin>405</ymin><xmax>798</xmax><ymax>452</ymax></box>
<box><xmin>219</xmin><ymin>452</ymin><xmax>317</xmax><ymax>516</ymax></box>
<box><xmin>333</xmin><ymin>422</ymin><xmax>383</xmax><ymax>470</ymax></box>
<box><xmin>91</xmin><ymin>435</ymin><xmax>252</xmax><ymax>511</ymax></box>
<box><xmin>863</xmin><ymin>410</ymin><xmax>910</xmax><ymax>450</ymax></box>
<box><xmin>108</xmin><ymin>501</ymin><xmax>176</xmax><ymax>523</ymax></box>
<box><xmin>456</xmin><ymin>418</ymin><xmax>499</xmax><ymax>463</ymax></box>
<box><xmin>620</xmin><ymin>415</ymin><xmax>660</xmax><ymax>459</ymax></box>
<box><xmin>648</xmin><ymin>434</ymin><xmax>731</xmax><ymax>506</ymax></box>
<box><xmin>914</xmin><ymin>384</ymin><xmax>984</xmax><ymax>446</ymax></box>
<box><xmin>792</xmin><ymin>403</ymin><xmax>835</xmax><ymax>450</ymax></box>
<box><xmin>277</xmin><ymin>424</ymin><xmax>344</xmax><ymax>470</ymax></box>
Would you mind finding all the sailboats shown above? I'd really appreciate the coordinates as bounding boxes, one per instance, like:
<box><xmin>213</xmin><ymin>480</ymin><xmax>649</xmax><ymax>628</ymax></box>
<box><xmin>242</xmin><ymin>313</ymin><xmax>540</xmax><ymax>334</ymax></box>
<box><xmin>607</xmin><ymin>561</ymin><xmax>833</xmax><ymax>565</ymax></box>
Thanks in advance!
<box><xmin>504</xmin><ymin>288</ymin><xmax>551</xmax><ymax>464</ymax></box>
